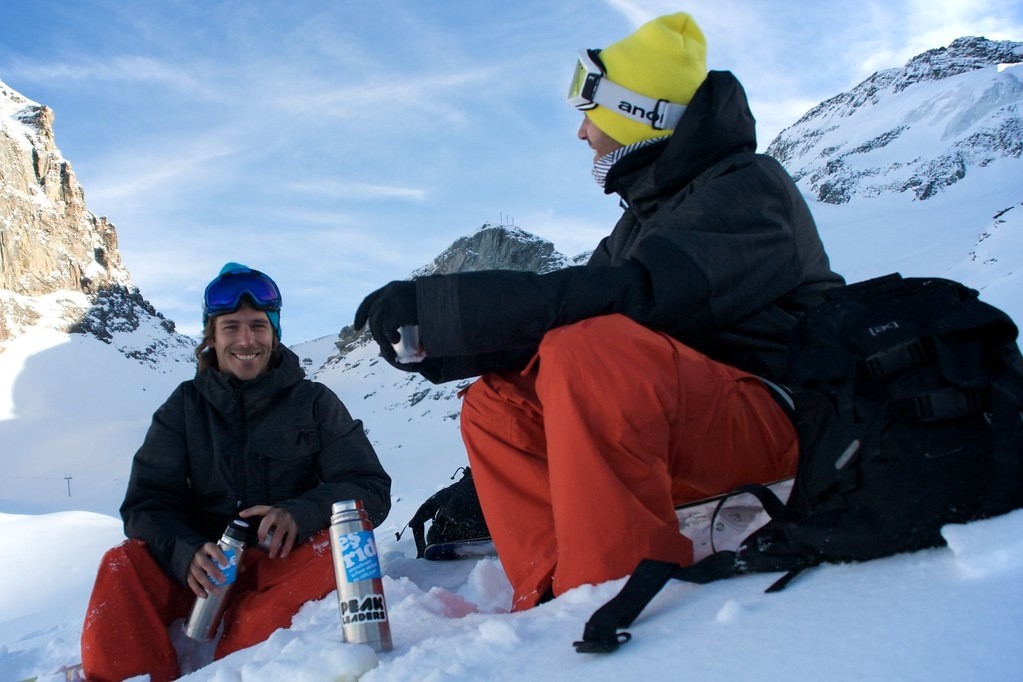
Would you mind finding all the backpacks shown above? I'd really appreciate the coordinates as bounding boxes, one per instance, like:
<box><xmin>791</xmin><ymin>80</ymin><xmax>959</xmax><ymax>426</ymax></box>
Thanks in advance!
<box><xmin>570</xmin><ymin>270</ymin><xmax>1023</xmax><ymax>652</ymax></box>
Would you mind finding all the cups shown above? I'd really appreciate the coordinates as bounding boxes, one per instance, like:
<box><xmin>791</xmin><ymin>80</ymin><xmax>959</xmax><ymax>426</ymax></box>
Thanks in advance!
<box><xmin>391</xmin><ymin>323</ymin><xmax>425</xmax><ymax>363</ymax></box>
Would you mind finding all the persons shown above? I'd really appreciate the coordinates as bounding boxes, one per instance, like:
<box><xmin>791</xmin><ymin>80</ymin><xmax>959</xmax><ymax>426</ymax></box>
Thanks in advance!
<box><xmin>351</xmin><ymin>11</ymin><xmax>850</xmax><ymax>612</ymax></box>
<box><xmin>82</xmin><ymin>264</ymin><xmax>392</xmax><ymax>682</ymax></box>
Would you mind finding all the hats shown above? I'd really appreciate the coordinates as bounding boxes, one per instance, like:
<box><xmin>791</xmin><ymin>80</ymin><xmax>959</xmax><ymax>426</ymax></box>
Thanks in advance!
<box><xmin>202</xmin><ymin>262</ymin><xmax>282</xmax><ymax>344</ymax></box>
<box><xmin>583</xmin><ymin>11</ymin><xmax>708</xmax><ymax>145</ymax></box>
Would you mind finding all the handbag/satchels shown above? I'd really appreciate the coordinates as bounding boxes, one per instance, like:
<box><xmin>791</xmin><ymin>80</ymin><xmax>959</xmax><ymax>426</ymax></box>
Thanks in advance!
<box><xmin>396</xmin><ymin>466</ymin><xmax>497</xmax><ymax>560</ymax></box>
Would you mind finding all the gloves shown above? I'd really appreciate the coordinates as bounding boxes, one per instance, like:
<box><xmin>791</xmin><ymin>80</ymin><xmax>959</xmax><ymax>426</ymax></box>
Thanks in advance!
<box><xmin>353</xmin><ymin>280</ymin><xmax>421</xmax><ymax>359</ymax></box>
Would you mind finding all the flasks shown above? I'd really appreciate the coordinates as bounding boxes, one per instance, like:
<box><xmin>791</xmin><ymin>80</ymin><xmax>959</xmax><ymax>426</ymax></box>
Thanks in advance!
<box><xmin>330</xmin><ymin>499</ymin><xmax>392</xmax><ymax>651</ymax></box>
<box><xmin>180</xmin><ymin>520</ymin><xmax>250</xmax><ymax>642</ymax></box>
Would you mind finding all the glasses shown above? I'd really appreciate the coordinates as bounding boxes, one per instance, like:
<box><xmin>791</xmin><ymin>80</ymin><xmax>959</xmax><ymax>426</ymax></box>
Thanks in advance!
<box><xmin>204</xmin><ymin>269</ymin><xmax>281</xmax><ymax>310</ymax></box>
<box><xmin>566</xmin><ymin>48</ymin><xmax>607</xmax><ymax>111</ymax></box>
